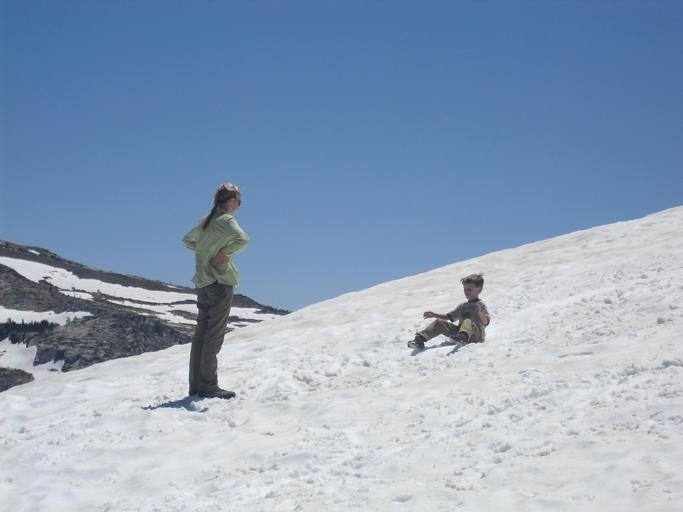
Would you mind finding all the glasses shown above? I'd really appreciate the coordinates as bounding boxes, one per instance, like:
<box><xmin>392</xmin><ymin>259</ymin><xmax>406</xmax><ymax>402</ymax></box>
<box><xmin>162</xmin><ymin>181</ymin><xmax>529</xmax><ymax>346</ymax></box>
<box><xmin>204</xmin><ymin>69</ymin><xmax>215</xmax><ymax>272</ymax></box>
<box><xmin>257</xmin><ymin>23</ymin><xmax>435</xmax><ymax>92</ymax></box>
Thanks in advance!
<box><xmin>233</xmin><ymin>199</ymin><xmax>242</xmax><ymax>203</ymax></box>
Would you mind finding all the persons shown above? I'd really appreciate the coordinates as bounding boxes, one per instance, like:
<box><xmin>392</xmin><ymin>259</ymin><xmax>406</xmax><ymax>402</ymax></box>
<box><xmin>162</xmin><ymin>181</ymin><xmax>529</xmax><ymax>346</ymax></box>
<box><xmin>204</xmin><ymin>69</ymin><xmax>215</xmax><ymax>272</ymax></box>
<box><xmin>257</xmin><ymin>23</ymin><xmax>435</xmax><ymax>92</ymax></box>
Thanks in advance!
<box><xmin>182</xmin><ymin>183</ymin><xmax>250</xmax><ymax>399</ymax></box>
<box><xmin>408</xmin><ymin>274</ymin><xmax>490</xmax><ymax>348</ymax></box>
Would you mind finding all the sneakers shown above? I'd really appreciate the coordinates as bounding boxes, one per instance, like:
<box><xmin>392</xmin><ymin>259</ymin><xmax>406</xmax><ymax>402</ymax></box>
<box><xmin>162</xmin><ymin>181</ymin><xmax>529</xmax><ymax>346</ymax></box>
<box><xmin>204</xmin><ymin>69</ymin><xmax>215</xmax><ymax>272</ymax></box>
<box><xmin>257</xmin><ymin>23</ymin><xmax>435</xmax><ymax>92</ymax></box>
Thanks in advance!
<box><xmin>190</xmin><ymin>388</ymin><xmax>237</xmax><ymax>399</ymax></box>
<box><xmin>449</xmin><ymin>334</ymin><xmax>467</xmax><ymax>344</ymax></box>
<box><xmin>407</xmin><ymin>335</ymin><xmax>424</xmax><ymax>348</ymax></box>
